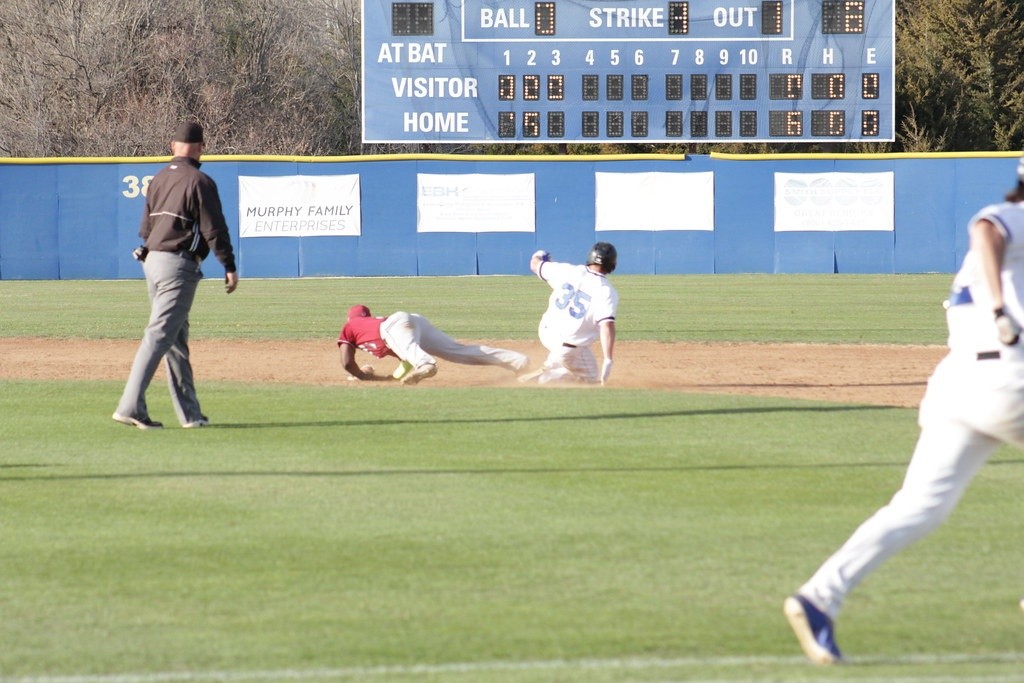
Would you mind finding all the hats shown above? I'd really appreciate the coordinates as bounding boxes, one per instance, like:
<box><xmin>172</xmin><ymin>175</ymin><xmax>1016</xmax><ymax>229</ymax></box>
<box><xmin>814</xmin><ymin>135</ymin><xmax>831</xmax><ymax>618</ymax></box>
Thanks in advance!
<box><xmin>349</xmin><ymin>305</ymin><xmax>372</xmax><ymax>320</ymax></box>
<box><xmin>174</xmin><ymin>121</ymin><xmax>206</xmax><ymax>146</ymax></box>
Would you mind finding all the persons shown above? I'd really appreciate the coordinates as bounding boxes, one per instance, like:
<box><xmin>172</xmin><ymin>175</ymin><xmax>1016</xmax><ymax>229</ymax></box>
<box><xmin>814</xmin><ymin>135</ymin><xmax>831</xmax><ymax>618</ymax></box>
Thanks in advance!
<box><xmin>518</xmin><ymin>243</ymin><xmax>620</xmax><ymax>387</ymax></box>
<box><xmin>338</xmin><ymin>305</ymin><xmax>529</xmax><ymax>384</ymax></box>
<box><xmin>107</xmin><ymin>123</ymin><xmax>240</xmax><ymax>432</ymax></box>
<box><xmin>783</xmin><ymin>148</ymin><xmax>1024</xmax><ymax>664</ymax></box>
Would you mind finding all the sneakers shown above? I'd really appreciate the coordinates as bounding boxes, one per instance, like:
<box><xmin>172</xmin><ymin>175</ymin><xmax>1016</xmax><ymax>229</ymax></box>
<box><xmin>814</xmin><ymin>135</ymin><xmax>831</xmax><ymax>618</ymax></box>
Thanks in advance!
<box><xmin>782</xmin><ymin>594</ymin><xmax>847</xmax><ymax>665</ymax></box>
<box><xmin>184</xmin><ymin>414</ymin><xmax>209</xmax><ymax>428</ymax></box>
<box><xmin>112</xmin><ymin>413</ymin><xmax>164</xmax><ymax>429</ymax></box>
<box><xmin>401</xmin><ymin>363</ymin><xmax>437</xmax><ymax>385</ymax></box>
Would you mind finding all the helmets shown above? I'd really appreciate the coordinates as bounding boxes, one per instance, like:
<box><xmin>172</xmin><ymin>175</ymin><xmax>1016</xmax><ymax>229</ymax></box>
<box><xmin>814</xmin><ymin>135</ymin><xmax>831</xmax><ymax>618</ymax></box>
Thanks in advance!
<box><xmin>587</xmin><ymin>240</ymin><xmax>618</xmax><ymax>274</ymax></box>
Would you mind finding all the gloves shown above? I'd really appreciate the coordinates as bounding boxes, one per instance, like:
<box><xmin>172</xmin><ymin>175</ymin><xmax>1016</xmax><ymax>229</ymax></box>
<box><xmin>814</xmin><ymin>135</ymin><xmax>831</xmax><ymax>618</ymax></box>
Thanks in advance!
<box><xmin>993</xmin><ymin>310</ymin><xmax>1022</xmax><ymax>348</ymax></box>
<box><xmin>601</xmin><ymin>358</ymin><xmax>613</xmax><ymax>385</ymax></box>
<box><xmin>532</xmin><ymin>250</ymin><xmax>551</xmax><ymax>262</ymax></box>
